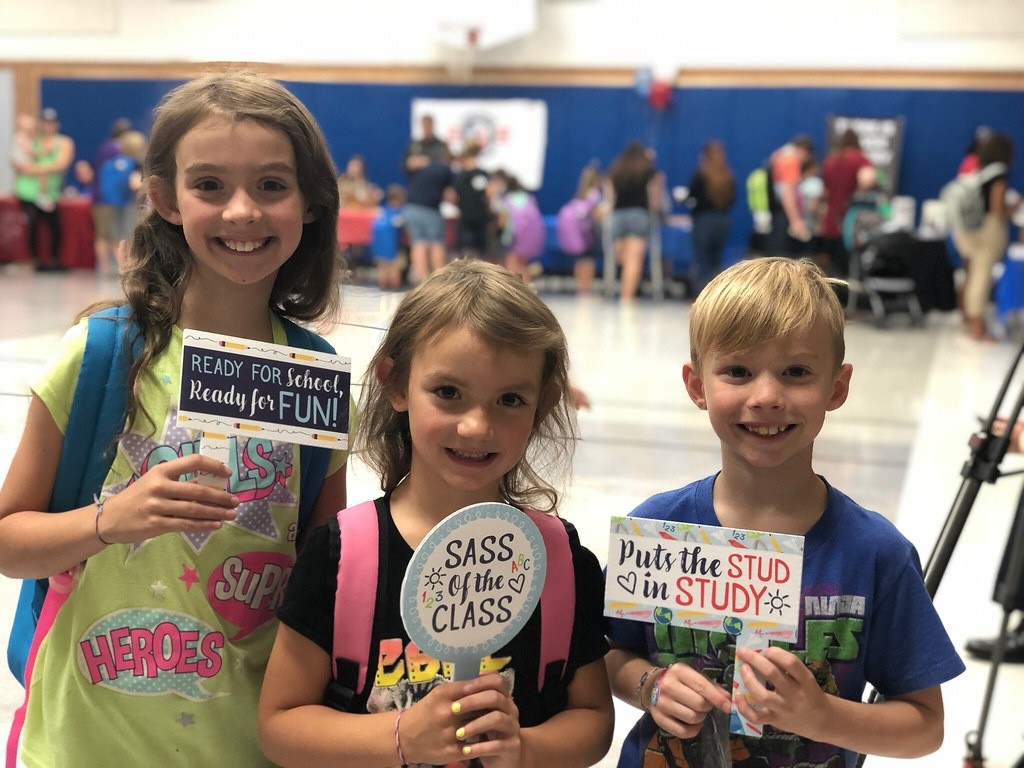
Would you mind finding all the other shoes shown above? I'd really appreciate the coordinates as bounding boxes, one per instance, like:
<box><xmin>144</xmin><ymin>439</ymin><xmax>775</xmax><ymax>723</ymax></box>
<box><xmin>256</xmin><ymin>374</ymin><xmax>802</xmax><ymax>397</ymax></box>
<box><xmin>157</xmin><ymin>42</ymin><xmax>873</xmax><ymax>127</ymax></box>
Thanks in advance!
<box><xmin>968</xmin><ymin>618</ymin><xmax>1024</xmax><ymax>661</ymax></box>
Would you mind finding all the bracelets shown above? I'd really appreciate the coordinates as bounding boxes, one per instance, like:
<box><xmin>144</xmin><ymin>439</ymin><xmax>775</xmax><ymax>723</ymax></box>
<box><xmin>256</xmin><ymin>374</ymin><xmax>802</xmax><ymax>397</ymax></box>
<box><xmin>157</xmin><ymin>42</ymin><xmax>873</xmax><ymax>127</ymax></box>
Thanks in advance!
<box><xmin>395</xmin><ymin>708</ymin><xmax>415</xmax><ymax>765</ymax></box>
<box><xmin>635</xmin><ymin>668</ymin><xmax>658</xmax><ymax>710</ymax></box>
<box><xmin>92</xmin><ymin>493</ymin><xmax>115</xmax><ymax>545</ymax></box>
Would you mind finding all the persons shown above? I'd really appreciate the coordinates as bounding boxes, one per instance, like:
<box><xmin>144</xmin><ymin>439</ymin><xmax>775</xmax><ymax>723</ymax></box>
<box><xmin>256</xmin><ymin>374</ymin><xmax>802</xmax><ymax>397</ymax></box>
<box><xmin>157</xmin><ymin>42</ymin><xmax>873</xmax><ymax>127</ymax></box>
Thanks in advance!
<box><xmin>687</xmin><ymin>141</ymin><xmax>735</xmax><ymax>304</ymax></box>
<box><xmin>748</xmin><ymin>132</ymin><xmax>876</xmax><ymax>312</ymax></box>
<box><xmin>12</xmin><ymin>109</ymin><xmax>74</xmax><ymax>273</ymax></box>
<box><xmin>77</xmin><ymin>117</ymin><xmax>149</xmax><ymax>280</ymax></box>
<box><xmin>964</xmin><ymin>417</ymin><xmax>1024</xmax><ymax>662</ymax></box>
<box><xmin>1</xmin><ymin>73</ymin><xmax>358</xmax><ymax>768</ymax></box>
<box><xmin>575</xmin><ymin>144</ymin><xmax>672</xmax><ymax>304</ymax></box>
<box><xmin>338</xmin><ymin>116</ymin><xmax>546</xmax><ymax>293</ymax></box>
<box><xmin>603</xmin><ymin>258</ymin><xmax>966</xmax><ymax>768</ymax></box>
<box><xmin>258</xmin><ymin>260</ymin><xmax>615</xmax><ymax>768</ymax></box>
<box><xmin>960</xmin><ymin>125</ymin><xmax>1024</xmax><ymax>340</ymax></box>
